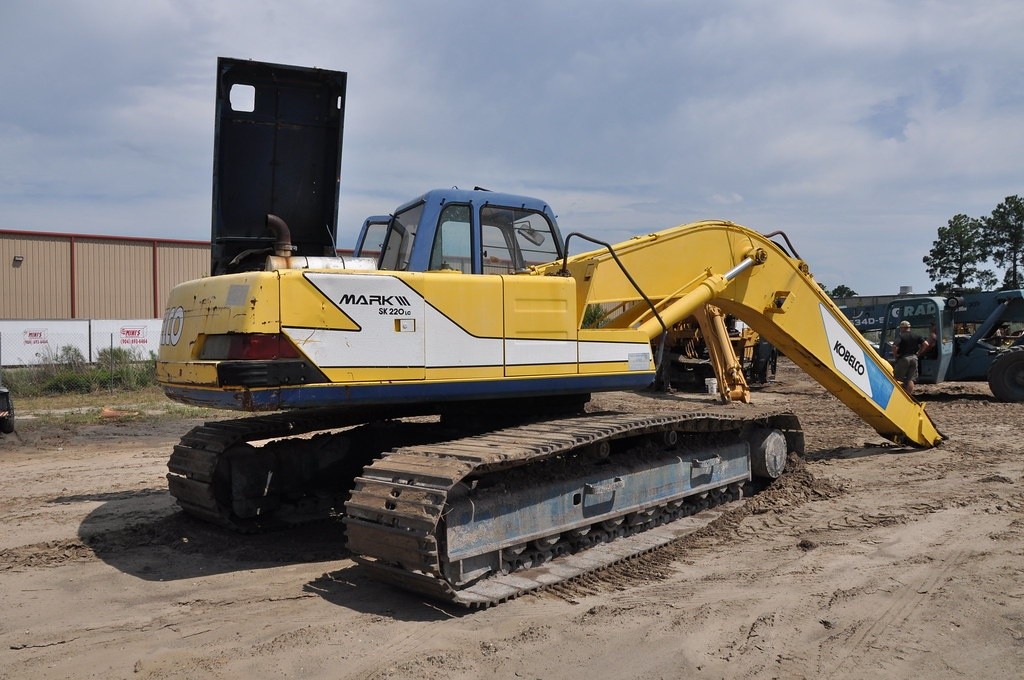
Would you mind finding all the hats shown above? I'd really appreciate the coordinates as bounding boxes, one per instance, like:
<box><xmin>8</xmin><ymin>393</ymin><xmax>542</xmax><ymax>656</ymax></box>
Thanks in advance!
<box><xmin>897</xmin><ymin>320</ymin><xmax>912</xmax><ymax>329</ymax></box>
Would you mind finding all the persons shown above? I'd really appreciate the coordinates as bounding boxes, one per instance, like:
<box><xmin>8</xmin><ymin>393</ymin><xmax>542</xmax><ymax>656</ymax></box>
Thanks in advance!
<box><xmin>926</xmin><ymin>323</ymin><xmax>938</xmax><ymax>349</ymax></box>
<box><xmin>891</xmin><ymin>320</ymin><xmax>930</xmax><ymax>395</ymax></box>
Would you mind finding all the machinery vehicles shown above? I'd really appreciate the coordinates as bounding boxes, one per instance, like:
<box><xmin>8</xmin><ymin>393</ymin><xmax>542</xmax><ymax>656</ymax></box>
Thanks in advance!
<box><xmin>157</xmin><ymin>186</ymin><xmax>951</xmax><ymax>610</ymax></box>
<box><xmin>841</xmin><ymin>290</ymin><xmax>1024</xmax><ymax>403</ymax></box>
<box><xmin>649</xmin><ymin>305</ymin><xmax>779</xmax><ymax>402</ymax></box>
